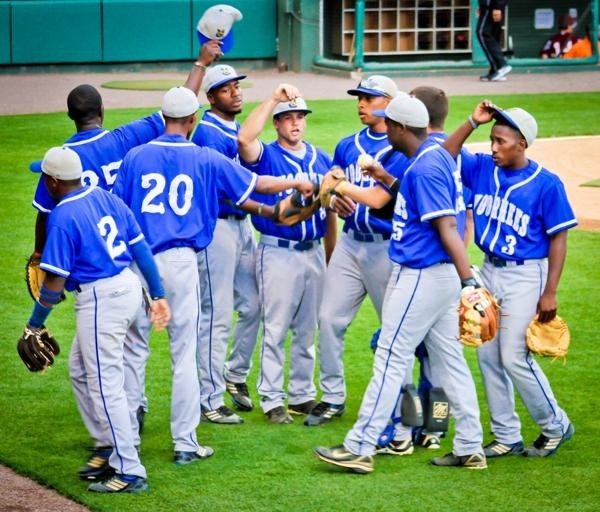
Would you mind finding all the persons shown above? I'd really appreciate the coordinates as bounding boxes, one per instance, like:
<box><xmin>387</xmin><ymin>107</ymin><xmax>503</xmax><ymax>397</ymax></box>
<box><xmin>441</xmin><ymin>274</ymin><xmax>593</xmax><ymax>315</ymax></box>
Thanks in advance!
<box><xmin>302</xmin><ymin>75</ymin><xmax>407</xmax><ymax>427</ymax></box>
<box><xmin>112</xmin><ymin>86</ymin><xmax>314</xmax><ymax>465</ymax></box>
<box><xmin>17</xmin><ymin>147</ymin><xmax>172</xmax><ymax>495</ymax></box>
<box><xmin>476</xmin><ymin>0</ymin><xmax>512</xmax><ymax>82</ymax></box>
<box><xmin>26</xmin><ymin>40</ymin><xmax>226</xmax><ymax>454</ymax></box>
<box><xmin>192</xmin><ymin>64</ymin><xmax>260</xmax><ymax>425</ymax></box>
<box><xmin>235</xmin><ymin>83</ymin><xmax>337</xmax><ymax>426</ymax></box>
<box><xmin>314</xmin><ymin>93</ymin><xmax>489</xmax><ymax>475</ymax></box>
<box><xmin>320</xmin><ymin>86</ymin><xmax>473</xmax><ymax>456</ymax></box>
<box><xmin>443</xmin><ymin>99</ymin><xmax>578</xmax><ymax>458</ymax></box>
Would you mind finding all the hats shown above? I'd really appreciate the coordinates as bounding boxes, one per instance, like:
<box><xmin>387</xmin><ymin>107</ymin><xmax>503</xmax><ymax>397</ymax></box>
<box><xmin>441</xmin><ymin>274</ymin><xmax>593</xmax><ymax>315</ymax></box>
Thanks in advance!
<box><xmin>273</xmin><ymin>96</ymin><xmax>312</xmax><ymax>118</ymax></box>
<box><xmin>347</xmin><ymin>75</ymin><xmax>399</xmax><ymax>99</ymax></box>
<box><xmin>162</xmin><ymin>86</ymin><xmax>203</xmax><ymax>118</ymax></box>
<box><xmin>371</xmin><ymin>95</ymin><xmax>429</xmax><ymax>128</ymax></box>
<box><xmin>30</xmin><ymin>147</ymin><xmax>83</xmax><ymax>180</ymax></box>
<box><xmin>487</xmin><ymin>103</ymin><xmax>538</xmax><ymax>148</ymax></box>
<box><xmin>196</xmin><ymin>5</ymin><xmax>243</xmax><ymax>52</ymax></box>
<box><xmin>202</xmin><ymin>65</ymin><xmax>248</xmax><ymax>92</ymax></box>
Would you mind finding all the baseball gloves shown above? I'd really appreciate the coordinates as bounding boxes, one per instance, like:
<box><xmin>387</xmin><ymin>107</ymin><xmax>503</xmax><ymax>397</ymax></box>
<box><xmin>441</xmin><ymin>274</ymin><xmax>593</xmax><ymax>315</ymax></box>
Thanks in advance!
<box><xmin>16</xmin><ymin>324</ymin><xmax>61</xmax><ymax>374</ymax></box>
<box><xmin>24</xmin><ymin>256</ymin><xmax>68</xmax><ymax>305</ymax></box>
<box><xmin>457</xmin><ymin>285</ymin><xmax>503</xmax><ymax>348</ymax></box>
<box><xmin>524</xmin><ymin>311</ymin><xmax>573</xmax><ymax>360</ymax></box>
<box><xmin>274</xmin><ymin>191</ymin><xmax>320</xmax><ymax>228</ymax></box>
<box><xmin>319</xmin><ymin>165</ymin><xmax>347</xmax><ymax>210</ymax></box>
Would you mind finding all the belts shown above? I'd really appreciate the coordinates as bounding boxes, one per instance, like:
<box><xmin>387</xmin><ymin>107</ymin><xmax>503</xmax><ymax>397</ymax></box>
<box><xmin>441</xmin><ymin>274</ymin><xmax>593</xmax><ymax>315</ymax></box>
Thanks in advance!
<box><xmin>216</xmin><ymin>209</ymin><xmax>248</xmax><ymax>221</ymax></box>
<box><xmin>262</xmin><ymin>236</ymin><xmax>323</xmax><ymax>253</ymax></box>
<box><xmin>484</xmin><ymin>254</ymin><xmax>527</xmax><ymax>270</ymax></box>
<box><xmin>341</xmin><ymin>226</ymin><xmax>391</xmax><ymax>243</ymax></box>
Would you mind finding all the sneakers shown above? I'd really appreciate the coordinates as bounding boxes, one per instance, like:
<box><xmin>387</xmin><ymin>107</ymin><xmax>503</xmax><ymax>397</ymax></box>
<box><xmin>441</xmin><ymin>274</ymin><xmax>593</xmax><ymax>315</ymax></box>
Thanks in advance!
<box><xmin>482</xmin><ymin>435</ymin><xmax>525</xmax><ymax>458</ymax></box>
<box><xmin>430</xmin><ymin>445</ymin><xmax>489</xmax><ymax>470</ymax></box>
<box><xmin>314</xmin><ymin>443</ymin><xmax>375</xmax><ymax>474</ymax></box>
<box><xmin>223</xmin><ymin>374</ymin><xmax>253</xmax><ymax>411</ymax></box>
<box><xmin>200</xmin><ymin>399</ymin><xmax>243</xmax><ymax>424</ymax></box>
<box><xmin>524</xmin><ymin>423</ymin><xmax>575</xmax><ymax>458</ymax></box>
<box><xmin>77</xmin><ymin>447</ymin><xmax>114</xmax><ymax>478</ymax></box>
<box><xmin>175</xmin><ymin>442</ymin><xmax>214</xmax><ymax>464</ymax></box>
<box><xmin>374</xmin><ymin>433</ymin><xmax>415</xmax><ymax>456</ymax></box>
<box><xmin>287</xmin><ymin>398</ymin><xmax>316</xmax><ymax>415</ymax></box>
<box><xmin>87</xmin><ymin>471</ymin><xmax>150</xmax><ymax>495</ymax></box>
<box><xmin>304</xmin><ymin>397</ymin><xmax>345</xmax><ymax>427</ymax></box>
<box><xmin>489</xmin><ymin>64</ymin><xmax>512</xmax><ymax>81</ymax></box>
<box><xmin>419</xmin><ymin>431</ymin><xmax>440</xmax><ymax>450</ymax></box>
<box><xmin>479</xmin><ymin>74</ymin><xmax>508</xmax><ymax>83</ymax></box>
<box><xmin>265</xmin><ymin>404</ymin><xmax>294</xmax><ymax>425</ymax></box>
